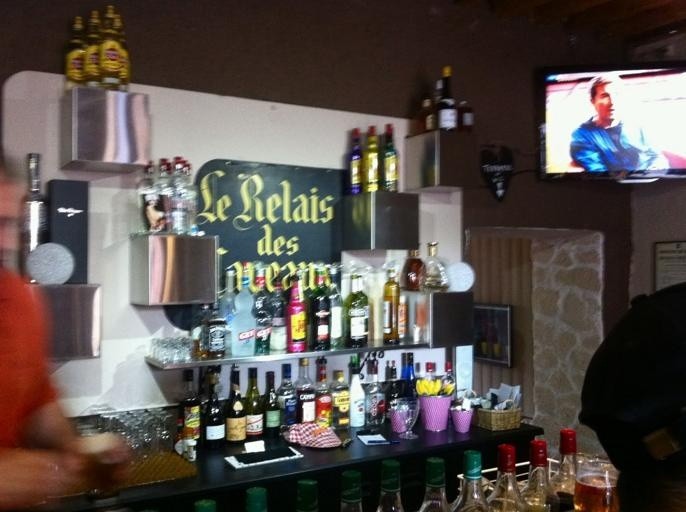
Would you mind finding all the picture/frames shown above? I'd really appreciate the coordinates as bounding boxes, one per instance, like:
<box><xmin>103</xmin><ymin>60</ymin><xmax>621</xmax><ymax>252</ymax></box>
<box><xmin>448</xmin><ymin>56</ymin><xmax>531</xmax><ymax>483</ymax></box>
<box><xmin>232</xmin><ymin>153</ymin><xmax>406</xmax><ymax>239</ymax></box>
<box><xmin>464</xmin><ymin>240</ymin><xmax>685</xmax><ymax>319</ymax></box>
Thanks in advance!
<box><xmin>472</xmin><ymin>302</ymin><xmax>514</xmax><ymax>369</ymax></box>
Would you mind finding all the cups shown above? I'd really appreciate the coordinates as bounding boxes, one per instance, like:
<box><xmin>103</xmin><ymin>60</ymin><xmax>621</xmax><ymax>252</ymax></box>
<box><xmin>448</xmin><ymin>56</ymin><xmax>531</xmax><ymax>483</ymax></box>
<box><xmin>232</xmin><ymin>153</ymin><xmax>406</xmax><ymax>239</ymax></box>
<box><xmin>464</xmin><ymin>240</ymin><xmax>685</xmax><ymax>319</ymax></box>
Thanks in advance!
<box><xmin>78</xmin><ymin>404</ymin><xmax>178</xmax><ymax>466</ymax></box>
<box><xmin>397</xmin><ymin>397</ymin><xmax>420</xmax><ymax>440</ymax></box>
<box><xmin>149</xmin><ymin>337</ymin><xmax>195</xmax><ymax>367</ymax></box>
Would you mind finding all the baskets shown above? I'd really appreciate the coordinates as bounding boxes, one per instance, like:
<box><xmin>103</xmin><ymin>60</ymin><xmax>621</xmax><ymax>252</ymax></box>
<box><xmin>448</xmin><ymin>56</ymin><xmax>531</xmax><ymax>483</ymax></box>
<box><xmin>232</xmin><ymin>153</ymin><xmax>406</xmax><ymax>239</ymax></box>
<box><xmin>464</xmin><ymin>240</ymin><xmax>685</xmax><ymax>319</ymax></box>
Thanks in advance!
<box><xmin>473</xmin><ymin>400</ymin><xmax>521</xmax><ymax>432</ymax></box>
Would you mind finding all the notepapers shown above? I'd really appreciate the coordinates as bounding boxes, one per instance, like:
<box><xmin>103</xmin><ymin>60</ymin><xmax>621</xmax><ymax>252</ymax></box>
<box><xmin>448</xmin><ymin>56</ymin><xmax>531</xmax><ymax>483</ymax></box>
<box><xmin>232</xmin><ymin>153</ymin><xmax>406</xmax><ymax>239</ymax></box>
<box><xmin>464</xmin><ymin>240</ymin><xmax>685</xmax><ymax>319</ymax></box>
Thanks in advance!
<box><xmin>357</xmin><ymin>434</ymin><xmax>389</xmax><ymax>445</ymax></box>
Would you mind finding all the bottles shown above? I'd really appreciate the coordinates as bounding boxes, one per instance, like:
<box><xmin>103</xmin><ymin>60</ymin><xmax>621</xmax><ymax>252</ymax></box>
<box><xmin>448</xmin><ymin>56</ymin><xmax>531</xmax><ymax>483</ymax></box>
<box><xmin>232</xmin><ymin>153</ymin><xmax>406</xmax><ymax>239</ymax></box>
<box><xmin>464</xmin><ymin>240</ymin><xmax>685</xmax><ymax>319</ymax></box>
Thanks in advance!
<box><xmin>252</xmin><ymin>271</ymin><xmax>272</xmax><ymax>355</ymax></box>
<box><xmin>287</xmin><ymin>273</ymin><xmax>307</xmax><ymax>352</ymax></box>
<box><xmin>193</xmin><ymin>498</ymin><xmax>215</xmax><ymax>512</ymax></box>
<box><xmin>231</xmin><ymin>269</ymin><xmax>257</xmax><ymax>356</ymax></box>
<box><xmin>325</xmin><ymin>268</ymin><xmax>345</xmax><ymax>347</ymax></box>
<box><xmin>203</xmin><ymin>365</ymin><xmax>226</xmax><ymax>454</ymax></box>
<box><xmin>381</xmin><ymin>125</ymin><xmax>399</xmax><ymax>188</ymax></box>
<box><xmin>269</xmin><ymin>277</ymin><xmax>288</xmax><ymax>350</ymax></box>
<box><xmin>85</xmin><ymin>11</ymin><xmax>100</xmax><ymax>83</ymax></box>
<box><xmin>437</xmin><ymin>65</ymin><xmax>459</xmax><ymax>128</ymax></box>
<box><xmin>423</xmin><ymin>241</ymin><xmax>449</xmax><ymax>291</ymax></box>
<box><xmin>276</xmin><ymin>364</ymin><xmax>296</xmax><ymax>425</ymax></box>
<box><xmin>260</xmin><ymin>371</ymin><xmax>281</xmax><ymax>436</ymax></box>
<box><xmin>309</xmin><ymin>269</ymin><xmax>330</xmax><ymax>351</ymax></box>
<box><xmin>404</xmin><ymin>244</ymin><xmax>426</xmax><ymax>291</ymax></box>
<box><xmin>366</xmin><ymin>360</ymin><xmax>385</xmax><ymax>423</ymax></box>
<box><xmin>338</xmin><ymin>470</ymin><xmax>363</xmax><ymax>512</ymax></box>
<box><xmin>293</xmin><ymin>358</ymin><xmax>316</xmax><ymax>422</ymax></box>
<box><xmin>348</xmin><ymin>129</ymin><xmax>363</xmax><ymax>194</ymax></box>
<box><xmin>180</xmin><ymin>369</ymin><xmax>202</xmax><ymax>441</ymax></box>
<box><xmin>18</xmin><ymin>154</ymin><xmax>50</xmax><ymax>282</ymax></box>
<box><xmin>357</xmin><ymin>275</ymin><xmax>369</xmax><ymax>320</ymax></box>
<box><xmin>209</xmin><ymin>303</ymin><xmax>226</xmax><ymax>358</ymax></box>
<box><xmin>401</xmin><ymin>353</ymin><xmax>406</xmax><ymax>382</ymax></box>
<box><xmin>100</xmin><ymin>5</ymin><xmax>120</xmax><ymax>89</ymax></box>
<box><xmin>441</xmin><ymin>362</ymin><xmax>456</xmax><ymax>402</ymax></box>
<box><xmin>519</xmin><ymin>439</ymin><xmax>559</xmax><ymax>511</ymax></box>
<box><xmin>245</xmin><ymin>486</ymin><xmax>268</xmax><ymax>512</ymax></box>
<box><xmin>363</xmin><ymin>126</ymin><xmax>377</xmax><ymax>188</ymax></box>
<box><xmin>115</xmin><ymin>14</ymin><xmax>130</xmax><ymax>85</ymax></box>
<box><xmin>421</xmin><ymin>90</ymin><xmax>436</xmax><ymax>131</ymax></box>
<box><xmin>244</xmin><ymin>368</ymin><xmax>263</xmax><ymax>440</ymax></box>
<box><xmin>192</xmin><ymin>302</ymin><xmax>210</xmax><ymax>361</ymax></box>
<box><xmin>383</xmin><ymin>268</ymin><xmax>400</xmax><ymax>344</ymax></box>
<box><xmin>487</xmin><ymin>444</ymin><xmax>529</xmax><ymax>511</ymax></box>
<box><xmin>296</xmin><ymin>478</ymin><xmax>317</xmax><ymax>511</ymax></box>
<box><xmin>329</xmin><ymin>369</ymin><xmax>349</xmax><ymax>426</ymax></box>
<box><xmin>139</xmin><ymin>160</ymin><xmax>160</xmax><ymax>232</ymax></box>
<box><xmin>315</xmin><ymin>357</ymin><xmax>332</xmax><ymax>428</ymax></box>
<box><xmin>435</xmin><ymin>78</ymin><xmax>443</xmax><ymax>111</ymax></box>
<box><xmin>404</xmin><ymin>352</ymin><xmax>418</xmax><ymax>401</ymax></box>
<box><xmin>385</xmin><ymin>367</ymin><xmax>401</xmax><ymax>421</ymax></box>
<box><xmin>65</xmin><ymin>16</ymin><xmax>86</xmax><ymax>87</ymax></box>
<box><xmin>349</xmin><ymin>367</ymin><xmax>366</xmax><ymax>428</ymax></box>
<box><xmin>171</xmin><ymin>159</ymin><xmax>190</xmax><ymax>234</ymax></box>
<box><xmin>344</xmin><ymin>280</ymin><xmax>368</xmax><ymax>348</ymax></box>
<box><xmin>550</xmin><ymin>429</ymin><xmax>580</xmax><ymax>504</ymax></box>
<box><xmin>223</xmin><ymin>367</ymin><xmax>246</xmax><ymax>446</ymax></box>
<box><xmin>376</xmin><ymin>458</ymin><xmax>403</xmax><ymax>511</ymax></box>
<box><xmin>425</xmin><ymin>363</ymin><xmax>437</xmax><ymax>383</ymax></box>
<box><xmin>453</xmin><ymin>450</ymin><xmax>490</xmax><ymax>512</ymax></box>
<box><xmin>417</xmin><ymin>455</ymin><xmax>450</xmax><ymax>512</ymax></box>
<box><xmin>218</xmin><ymin>264</ymin><xmax>236</xmax><ymax>356</ymax></box>
<box><xmin>156</xmin><ymin>156</ymin><xmax>197</xmax><ymax>234</ymax></box>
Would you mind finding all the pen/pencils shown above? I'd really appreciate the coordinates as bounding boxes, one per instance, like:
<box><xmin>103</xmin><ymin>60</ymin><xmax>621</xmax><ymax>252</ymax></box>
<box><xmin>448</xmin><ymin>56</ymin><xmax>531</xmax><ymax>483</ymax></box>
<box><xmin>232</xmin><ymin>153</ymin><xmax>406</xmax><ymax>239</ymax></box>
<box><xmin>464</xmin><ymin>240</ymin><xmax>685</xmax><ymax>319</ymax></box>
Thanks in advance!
<box><xmin>367</xmin><ymin>440</ymin><xmax>401</xmax><ymax>444</ymax></box>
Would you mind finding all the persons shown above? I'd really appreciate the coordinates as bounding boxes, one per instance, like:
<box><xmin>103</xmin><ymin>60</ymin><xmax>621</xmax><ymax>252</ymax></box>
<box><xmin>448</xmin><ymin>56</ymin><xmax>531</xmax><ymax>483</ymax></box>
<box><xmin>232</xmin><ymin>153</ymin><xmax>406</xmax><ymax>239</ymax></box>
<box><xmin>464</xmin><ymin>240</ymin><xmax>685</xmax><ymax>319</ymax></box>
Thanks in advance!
<box><xmin>0</xmin><ymin>153</ymin><xmax>131</xmax><ymax>511</ymax></box>
<box><xmin>579</xmin><ymin>280</ymin><xmax>686</xmax><ymax>511</ymax></box>
<box><xmin>569</xmin><ymin>74</ymin><xmax>671</xmax><ymax>172</ymax></box>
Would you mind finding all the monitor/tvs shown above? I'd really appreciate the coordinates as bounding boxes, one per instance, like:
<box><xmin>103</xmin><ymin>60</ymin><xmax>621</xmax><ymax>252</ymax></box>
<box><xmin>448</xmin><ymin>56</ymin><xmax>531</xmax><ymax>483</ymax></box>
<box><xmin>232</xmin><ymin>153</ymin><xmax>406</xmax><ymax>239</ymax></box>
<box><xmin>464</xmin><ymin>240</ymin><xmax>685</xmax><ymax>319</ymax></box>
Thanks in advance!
<box><xmin>536</xmin><ymin>62</ymin><xmax>686</xmax><ymax>179</ymax></box>
